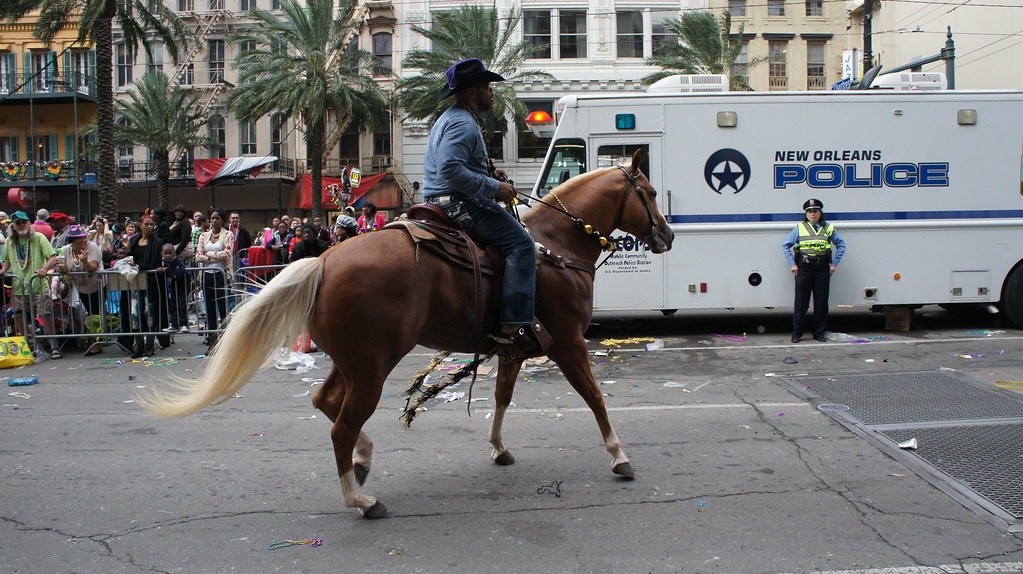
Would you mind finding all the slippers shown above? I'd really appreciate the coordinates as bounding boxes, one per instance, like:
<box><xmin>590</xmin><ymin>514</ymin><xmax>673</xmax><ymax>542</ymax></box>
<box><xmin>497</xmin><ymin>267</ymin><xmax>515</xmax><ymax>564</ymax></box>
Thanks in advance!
<box><xmin>84</xmin><ymin>348</ymin><xmax>103</xmax><ymax>356</ymax></box>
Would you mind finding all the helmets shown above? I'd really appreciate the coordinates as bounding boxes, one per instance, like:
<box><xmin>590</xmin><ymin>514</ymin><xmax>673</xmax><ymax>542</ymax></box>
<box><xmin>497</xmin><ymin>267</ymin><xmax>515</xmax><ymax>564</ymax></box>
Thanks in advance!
<box><xmin>336</xmin><ymin>215</ymin><xmax>359</xmax><ymax>228</ymax></box>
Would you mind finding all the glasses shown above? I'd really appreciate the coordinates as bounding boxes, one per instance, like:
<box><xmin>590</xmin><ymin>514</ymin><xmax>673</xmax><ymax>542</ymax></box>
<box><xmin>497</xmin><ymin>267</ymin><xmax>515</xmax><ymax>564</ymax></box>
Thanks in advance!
<box><xmin>336</xmin><ymin>223</ymin><xmax>345</xmax><ymax>229</ymax></box>
<box><xmin>14</xmin><ymin>220</ymin><xmax>29</xmax><ymax>225</ymax></box>
<box><xmin>470</xmin><ymin>80</ymin><xmax>490</xmax><ymax>91</ymax></box>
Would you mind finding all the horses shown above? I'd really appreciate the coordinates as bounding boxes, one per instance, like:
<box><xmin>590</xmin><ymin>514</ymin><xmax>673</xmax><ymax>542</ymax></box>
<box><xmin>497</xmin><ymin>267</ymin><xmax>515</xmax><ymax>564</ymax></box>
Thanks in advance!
<box><xmin>133</xmin><ymin>146</ymin><xmax>677</xmax><ymax>521</ymax></box>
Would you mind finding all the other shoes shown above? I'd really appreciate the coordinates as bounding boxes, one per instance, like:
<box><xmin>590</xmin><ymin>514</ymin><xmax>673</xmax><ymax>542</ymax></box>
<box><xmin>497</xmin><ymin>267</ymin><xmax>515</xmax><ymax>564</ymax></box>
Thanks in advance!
<box><xmin>791</xmin><ymin>334</ymin><xmax>799</xmax><ymax>343</ymax></box>
<box><xmin>116</xmin><ymin>324</ymin><xmax>189</xmax><ymax>359</ymax></box>
<box><xmin>813</xmin><ymin>334</ymin><xmax>825</xmax><ymax>342</ymax></box>
<box><xmin>205</xmin><ymin>335</ymin><xmax>217</xmax><ymax>345</ymax></box>
<box><xmin>488</xmin><ymin>321</ymin><xmax>535</xmax><ymax>344</ymax></box>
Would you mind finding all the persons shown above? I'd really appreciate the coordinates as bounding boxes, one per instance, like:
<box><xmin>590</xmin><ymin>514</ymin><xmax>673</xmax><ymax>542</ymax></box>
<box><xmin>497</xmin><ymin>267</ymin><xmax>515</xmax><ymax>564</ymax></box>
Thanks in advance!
<box><xmin>422</xmin><ymin>57</ymin><xmax>540</xmax><ymax>349</ymax></box>
<box><xmin>0</xmin><ymin>203</ymin><xmax>251</xmax><ymax>360</ymax></box>
<box><xmin>255</xmin><ymin>202</ymin><xmax>386</xmax><ymax>285</ymax></box>
<box><xmin>782</xmin><ymin>199</ymin><xmax>846</xmax><ymax>343</ymax></box>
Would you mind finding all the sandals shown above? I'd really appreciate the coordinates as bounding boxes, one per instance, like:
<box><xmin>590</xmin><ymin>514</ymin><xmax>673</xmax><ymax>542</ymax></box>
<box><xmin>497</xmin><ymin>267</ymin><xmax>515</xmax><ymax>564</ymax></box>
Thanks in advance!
<box><xmin>50</xmin><ymin>347</ymin><xmax>62</xmax><ymax>359</ymax></box>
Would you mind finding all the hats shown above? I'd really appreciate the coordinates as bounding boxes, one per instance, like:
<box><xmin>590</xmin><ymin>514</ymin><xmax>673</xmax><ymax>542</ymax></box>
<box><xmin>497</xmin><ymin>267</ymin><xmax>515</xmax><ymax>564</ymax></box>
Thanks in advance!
<box><xmin>803</xmin><ymin>199</ymin><xmax>824</xmax><ymax>212</ymax></box>
<box><xmin>437</xmin><ymin>59</ymin><xmax>506</xmax><ymax>101</ymax></box>
<box><xmin>175</xmin><ymin>204</ymin><xmax>186</xmax><ymax>213</ymax></box>
<box><xmin>11</xmin><ymin>210</ymin><xmax>30</xmax><ymax>223</ymax></box>
<box><xmin>37</xmin><ymin>209</ymin><xmax>49</xmax><ymax>220</ymax></box>
<box><xmin>67</xmin><ymin>224</ymin><xmax>90</xmax><ymax>236</ymax></box>
<box><xmin>345</xmin><ymin>206</ymin><xmax>355</xmax><ymax>214</ymax></box>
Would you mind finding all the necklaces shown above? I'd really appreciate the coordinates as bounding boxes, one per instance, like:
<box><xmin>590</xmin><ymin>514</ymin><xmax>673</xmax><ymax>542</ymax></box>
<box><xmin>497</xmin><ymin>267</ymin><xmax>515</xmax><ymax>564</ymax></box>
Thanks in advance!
<box><xmin>15</xmin><ymin>239</ymin><xmax>28</xmax><ymax>270</ymax></box>
<box><xmin>211</xmin><ymin>232</ymin><xmax>220</xmax><ymax>238</ymax></box>
<box><xmin>229</xmin><ymin>223</ymin><xmax>239</xmax><ymax>241</ymax></box>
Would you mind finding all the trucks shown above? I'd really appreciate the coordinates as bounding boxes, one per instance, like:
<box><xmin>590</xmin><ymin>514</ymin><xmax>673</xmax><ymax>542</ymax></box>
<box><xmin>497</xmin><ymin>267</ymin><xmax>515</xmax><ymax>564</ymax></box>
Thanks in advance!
<box><xmin>512</xmin><ymin>74</ymin><xmax>1022</xmax><ymax>333</ymax></box>
